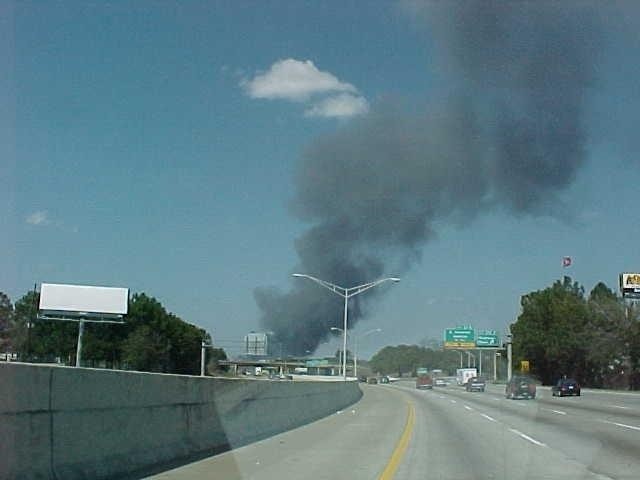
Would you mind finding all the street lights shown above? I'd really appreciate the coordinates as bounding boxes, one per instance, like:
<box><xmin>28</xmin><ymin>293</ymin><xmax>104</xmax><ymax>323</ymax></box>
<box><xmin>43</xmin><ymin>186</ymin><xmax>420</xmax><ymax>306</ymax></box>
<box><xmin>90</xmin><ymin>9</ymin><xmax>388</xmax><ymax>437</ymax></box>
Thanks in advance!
<box><xmin>291</xmin><ymin>273</ymin><xmax>400</xmax><ymax>375</ymax></box>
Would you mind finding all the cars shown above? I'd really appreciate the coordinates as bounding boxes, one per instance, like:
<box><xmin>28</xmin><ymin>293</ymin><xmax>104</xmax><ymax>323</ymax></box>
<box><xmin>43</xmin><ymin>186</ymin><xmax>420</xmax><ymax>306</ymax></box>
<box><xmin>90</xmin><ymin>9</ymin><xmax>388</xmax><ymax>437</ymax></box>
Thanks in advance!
<box><xmin>359</xmin><ymin>375</ymin><xmax>389</xmax><ymax>384</ymax></box>
<box><xmin>552</xmin><ymin>377</ymin><xmax>581</xmax><ymax>396</ymax></box>
<box><xmin>416</xmin><ymin>368</ymin><xmax>446</xmax><ymax>389</ymax></box>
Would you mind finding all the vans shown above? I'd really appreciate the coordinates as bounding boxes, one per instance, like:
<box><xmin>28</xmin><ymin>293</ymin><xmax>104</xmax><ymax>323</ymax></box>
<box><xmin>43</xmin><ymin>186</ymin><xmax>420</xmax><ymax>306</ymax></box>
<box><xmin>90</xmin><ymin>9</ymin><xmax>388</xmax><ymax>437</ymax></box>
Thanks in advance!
<box><xmin>455</xmin><ymin>368</ymin><xmax>477</xmax><ymax>385</ymax></box>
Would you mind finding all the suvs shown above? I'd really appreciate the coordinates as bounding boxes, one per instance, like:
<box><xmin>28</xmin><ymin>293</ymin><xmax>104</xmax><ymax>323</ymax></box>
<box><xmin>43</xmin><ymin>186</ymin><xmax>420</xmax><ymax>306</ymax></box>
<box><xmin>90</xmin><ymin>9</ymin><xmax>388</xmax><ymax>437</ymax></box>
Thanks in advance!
<box><xmin>504</xmin><ymin>376</ymin><xmax>536</xmax><ymax>399</ymax></box>
<box><xmin>467</xmin><ymin>377</ymin><xmax>485</xmax><ymax>391</ymax></box>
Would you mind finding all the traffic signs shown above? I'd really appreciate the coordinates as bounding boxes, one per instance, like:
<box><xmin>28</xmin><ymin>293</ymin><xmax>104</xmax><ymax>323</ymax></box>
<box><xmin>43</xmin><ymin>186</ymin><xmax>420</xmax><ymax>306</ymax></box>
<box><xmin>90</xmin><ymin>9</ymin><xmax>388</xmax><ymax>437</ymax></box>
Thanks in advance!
<box><xmin>477</xmin><ymin>329</ymin><xmax>499</xmax><ymax>346</ymax></box>
<box><xmin>443</xmin><ymin>328</ymin><xmax>473</xmax><ymax>343</ymax></box>
